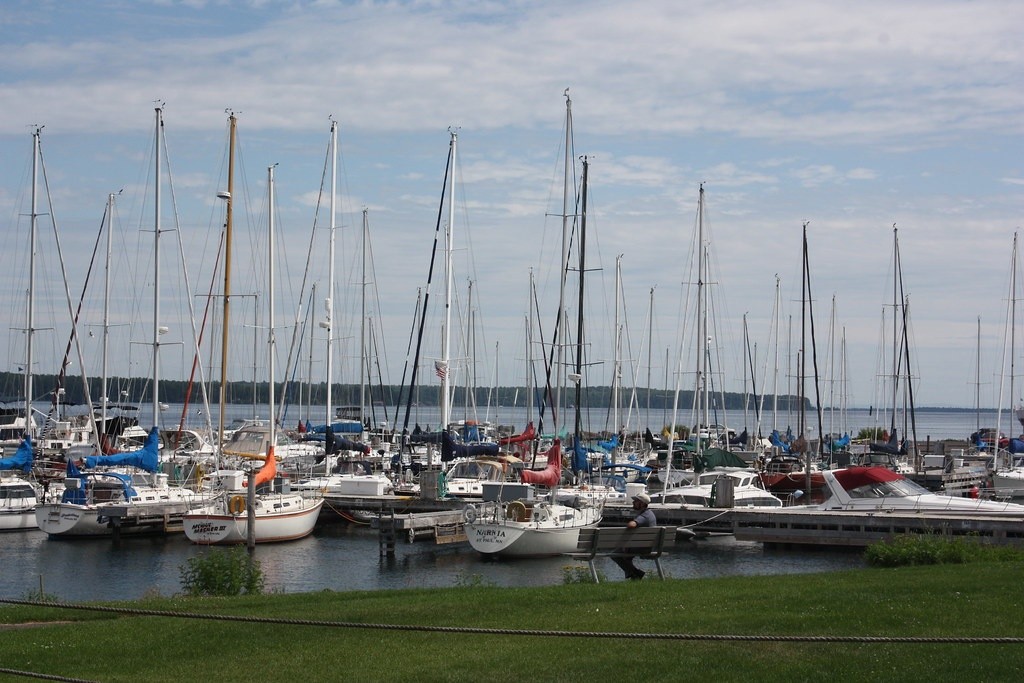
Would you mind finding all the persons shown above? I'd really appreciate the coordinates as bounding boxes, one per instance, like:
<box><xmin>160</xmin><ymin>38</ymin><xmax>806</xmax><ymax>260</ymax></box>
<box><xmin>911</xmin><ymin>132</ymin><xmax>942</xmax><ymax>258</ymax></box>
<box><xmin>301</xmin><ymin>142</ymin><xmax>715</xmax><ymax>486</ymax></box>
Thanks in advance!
<box><xmin>610</xmin><ymin>493</ymin><xmax>656</xmax><ymax>580</ymax></box>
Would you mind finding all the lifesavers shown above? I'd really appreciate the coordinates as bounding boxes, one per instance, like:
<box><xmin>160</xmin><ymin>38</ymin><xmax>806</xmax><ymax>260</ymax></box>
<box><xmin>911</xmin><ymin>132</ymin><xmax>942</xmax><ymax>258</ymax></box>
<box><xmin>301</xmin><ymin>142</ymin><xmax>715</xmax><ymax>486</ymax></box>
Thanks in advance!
<box><xmin>462</xmin><ymin>505</ymin><xmax>477</xmax><ymax>523</ymax></box>
<box><xmin>229</xmin><ymin>495</ymin><xmax>244</xmax><ymax>514</ymax></box>
<box><xmin>507</xmin><ymin>501</ymin><xmax>526</xmax><ymax>521</ymax></box>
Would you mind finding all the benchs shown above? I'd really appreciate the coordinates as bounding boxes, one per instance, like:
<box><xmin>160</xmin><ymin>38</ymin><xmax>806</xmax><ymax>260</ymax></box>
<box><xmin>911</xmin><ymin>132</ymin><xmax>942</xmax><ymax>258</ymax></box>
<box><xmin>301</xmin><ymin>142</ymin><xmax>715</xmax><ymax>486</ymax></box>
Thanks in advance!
<box><xmin>563</xmin><ymin>525</ymin><xmax>677</xmax><ymax>584</ymax></box>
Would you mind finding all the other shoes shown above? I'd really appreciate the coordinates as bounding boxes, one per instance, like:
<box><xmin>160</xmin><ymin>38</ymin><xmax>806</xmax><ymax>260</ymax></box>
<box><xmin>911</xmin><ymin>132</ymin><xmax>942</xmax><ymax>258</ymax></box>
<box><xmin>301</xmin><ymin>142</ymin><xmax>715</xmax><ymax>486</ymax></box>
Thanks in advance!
<box><xmin>630</xmin><ymin>569</ymin><xmax>645</xmax><ymax>581</ymax></box>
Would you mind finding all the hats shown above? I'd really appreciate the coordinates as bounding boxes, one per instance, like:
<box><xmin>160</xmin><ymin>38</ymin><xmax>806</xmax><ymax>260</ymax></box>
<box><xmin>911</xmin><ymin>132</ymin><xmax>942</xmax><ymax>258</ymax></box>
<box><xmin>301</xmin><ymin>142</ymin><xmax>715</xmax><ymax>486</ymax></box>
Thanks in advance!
<box><xmin>631</xmin><ymin>492</ymin><xmax>651</xmax><ymax>505</ymax></box>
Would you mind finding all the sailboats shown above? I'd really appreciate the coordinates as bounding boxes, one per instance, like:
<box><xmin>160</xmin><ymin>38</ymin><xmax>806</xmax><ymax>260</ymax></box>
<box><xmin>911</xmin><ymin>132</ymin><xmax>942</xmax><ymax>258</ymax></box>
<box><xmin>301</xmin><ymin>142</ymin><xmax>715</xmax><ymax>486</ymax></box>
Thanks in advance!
<box><xmin>0</xmin><ymin>85</ymin><xmax>1024</xmax><ymax>560</ymax></box>
